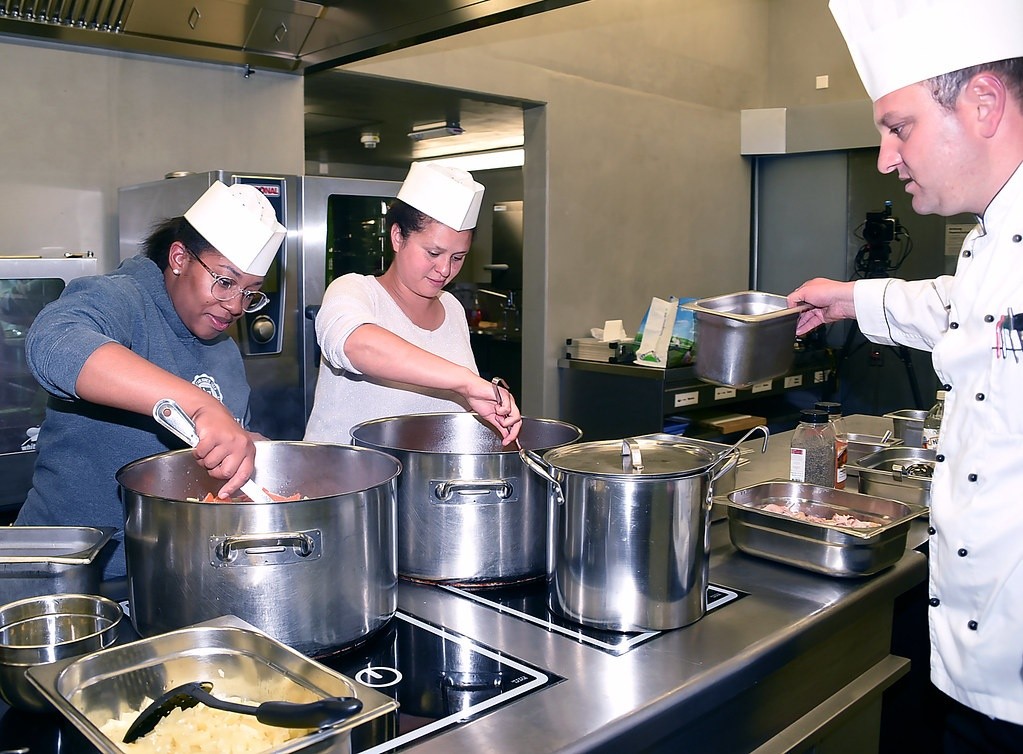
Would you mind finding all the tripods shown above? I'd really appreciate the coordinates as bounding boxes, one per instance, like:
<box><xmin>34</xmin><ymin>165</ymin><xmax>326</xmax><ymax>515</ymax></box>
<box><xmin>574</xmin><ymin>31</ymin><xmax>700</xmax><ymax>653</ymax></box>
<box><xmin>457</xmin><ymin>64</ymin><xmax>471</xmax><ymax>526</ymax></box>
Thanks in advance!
<box><xmin>822</xmin><ymin>241</ymin><xmax>924</xmax><ymax>411</ymax></box>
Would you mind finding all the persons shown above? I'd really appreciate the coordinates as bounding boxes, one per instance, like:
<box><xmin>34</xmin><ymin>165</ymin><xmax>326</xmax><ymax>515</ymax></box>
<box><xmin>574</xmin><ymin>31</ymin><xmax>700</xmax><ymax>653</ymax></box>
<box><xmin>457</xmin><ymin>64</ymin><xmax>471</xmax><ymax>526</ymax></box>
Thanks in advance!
<box><xmin>12</xmin><ymin>180</ymin><xmax>288</xmax><ymax>582</ymax></box>
<box><xmin>785</xmin><ymin>0</ymin><xmax>1023</xmax><ymax>754</ymax></box>
<box><xmin>296</xmin><ymin>162</ymin><xmax>522</xmax><ymax>445</ymax></box>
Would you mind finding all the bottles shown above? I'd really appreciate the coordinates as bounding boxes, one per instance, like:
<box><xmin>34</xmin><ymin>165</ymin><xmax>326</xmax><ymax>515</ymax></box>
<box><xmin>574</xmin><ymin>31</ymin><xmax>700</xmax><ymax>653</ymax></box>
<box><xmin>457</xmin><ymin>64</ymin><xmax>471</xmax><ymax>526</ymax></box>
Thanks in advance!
<box><xmin>470</xmin><ymin>299</ymin><xmax>481</xmax><ymax>328</ymax></box>
<box><xmin>813</xmin><ymin>402</ymin><xmax>849</xmax><ymax>490</ymax></box>
<box><xmin>921</xmin><ymin>390</ymin><xmax>947</xmax><ymax>450</ymax></box>
<box><xmin>789</xmin><ymin>410</ymin><xmax>835</xmax><ymax>489</ymax></box>
<box><xmin>500</xmin><ymin>292</ymin><xmax>515</xmax><ymax>335</ymax></box>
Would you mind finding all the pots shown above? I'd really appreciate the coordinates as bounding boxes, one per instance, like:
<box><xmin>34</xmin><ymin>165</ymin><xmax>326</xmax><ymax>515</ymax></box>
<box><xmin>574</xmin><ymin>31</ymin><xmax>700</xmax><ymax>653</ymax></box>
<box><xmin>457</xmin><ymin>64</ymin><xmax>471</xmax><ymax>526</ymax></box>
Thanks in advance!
<box><xmin>349</xmin><ymin>412</ymin><xmax>583</xmax><ymax>585</ymax></box>
<box><xmin>517</xmin><ymin>437</ymin><xmax>740</xmax><ymax>632</ymax></box>
<box><xmin>114</xmin><ymin>440</ymin><xmax>402</xmax><ymax>658</ymax></box>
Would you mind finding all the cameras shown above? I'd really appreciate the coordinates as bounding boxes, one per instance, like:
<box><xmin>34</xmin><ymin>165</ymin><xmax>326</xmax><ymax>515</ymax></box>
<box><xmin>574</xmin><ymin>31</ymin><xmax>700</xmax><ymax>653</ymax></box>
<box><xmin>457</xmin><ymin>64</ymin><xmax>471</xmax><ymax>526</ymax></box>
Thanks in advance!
<box><xmin>862</xmin><ymin>212</ymin><xmax>901</xmax><ymax>241</ymax></box>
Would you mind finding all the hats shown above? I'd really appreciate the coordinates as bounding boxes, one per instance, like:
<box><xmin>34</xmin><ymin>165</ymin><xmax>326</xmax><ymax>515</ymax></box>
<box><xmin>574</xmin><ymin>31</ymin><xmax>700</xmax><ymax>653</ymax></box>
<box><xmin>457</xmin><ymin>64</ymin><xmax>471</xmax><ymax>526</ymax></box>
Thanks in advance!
<box><xmin>822</xmin><ymin>0</ymin><xmax>1023</xmax><ymax>104</ymax></box>
<box><xmin>397</xmin><ymin>158</ymin><xmax>487</xmax><ymax>232</ymax></box>
<box><xmin>185</xmin><ymin>180</ymin><xmax>290</xmax><ymax>277</ymax></box>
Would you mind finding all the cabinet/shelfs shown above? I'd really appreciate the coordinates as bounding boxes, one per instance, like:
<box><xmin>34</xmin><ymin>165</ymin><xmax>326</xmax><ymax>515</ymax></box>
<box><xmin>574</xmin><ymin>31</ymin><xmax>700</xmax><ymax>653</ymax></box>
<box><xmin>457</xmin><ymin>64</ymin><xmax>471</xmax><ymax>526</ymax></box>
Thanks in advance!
<box><xmin>558</xmin><ymin>350</ymin><xmax>834</xmax><ymax>446</ymax></box>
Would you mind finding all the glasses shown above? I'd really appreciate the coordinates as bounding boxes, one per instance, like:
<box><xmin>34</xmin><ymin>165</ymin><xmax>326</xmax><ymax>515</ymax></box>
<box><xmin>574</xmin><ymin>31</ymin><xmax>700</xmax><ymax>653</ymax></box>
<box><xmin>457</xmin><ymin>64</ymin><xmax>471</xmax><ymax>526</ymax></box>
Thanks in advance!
<box><xmin>184</xmin><ymin>244</ymin><xmax>270</xmax><ymax>315</ymax></box>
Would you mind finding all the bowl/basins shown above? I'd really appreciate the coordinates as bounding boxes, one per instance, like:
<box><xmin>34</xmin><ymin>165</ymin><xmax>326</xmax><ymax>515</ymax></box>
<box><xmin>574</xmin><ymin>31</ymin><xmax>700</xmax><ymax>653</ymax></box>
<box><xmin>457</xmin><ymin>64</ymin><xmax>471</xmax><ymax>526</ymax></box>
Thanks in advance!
<box><xmin>837</xmin><ymin>433</ymin><xmax>904</xmax><ymax>476</ymax></box>
<box><xmin>678</xmin><ymin>290</ymin><xmax>813</xmax><ymax>388</ymax></box>
<box><xmin>0</xmin><ymin>594</ymin><xmax>125</xmax><ymax>709</ymax></box>
<box><xmin>21</xmin><ymin>612</ymin><xmax>400</xmax><ymax>754</ymax></box>
<box><xmin>0</xmin><ymin>523</ymin><xmax>117</xmax><ymax>605</ymax></box>
<box><xmin>712</xmin><ymin>477</ymin><xmax>931</xmax><ymax>580</ymax></box>
<box><xmin>883</xmin><ymin>409</ymin><xmax>930</xmax><ymax>448</ymax></box>
<box><xmin>842</xmin><ymin>446</ymin><xmax>936</xmax><ymax>518</ymax></box>
